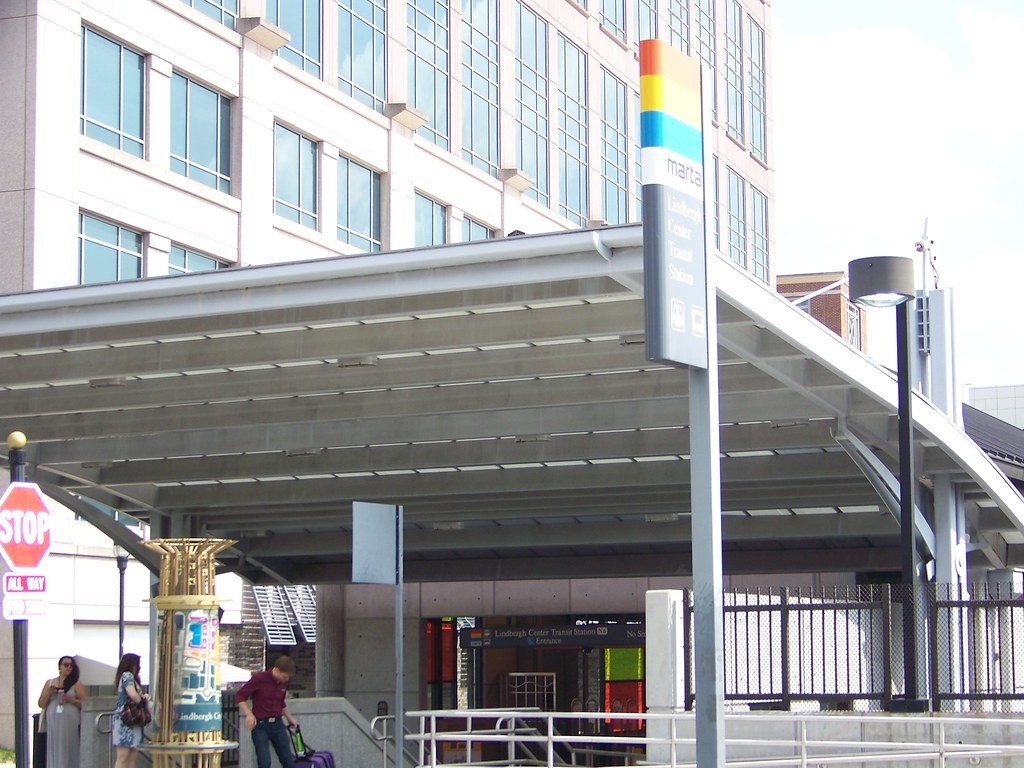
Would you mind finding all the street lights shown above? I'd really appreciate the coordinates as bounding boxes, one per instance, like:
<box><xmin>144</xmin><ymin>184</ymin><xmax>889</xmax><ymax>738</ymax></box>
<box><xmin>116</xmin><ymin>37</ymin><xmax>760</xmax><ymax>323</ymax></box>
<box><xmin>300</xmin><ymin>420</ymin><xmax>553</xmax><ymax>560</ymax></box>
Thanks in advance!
<box><xmin>848</xmin><ymin>255</ymin><xmax>929</xmax><ymax>713</ymax></box>
<box><xmin>113</xmin><ymin>542</ymin><xmax>131</xmax><ymax>663</ymax></box>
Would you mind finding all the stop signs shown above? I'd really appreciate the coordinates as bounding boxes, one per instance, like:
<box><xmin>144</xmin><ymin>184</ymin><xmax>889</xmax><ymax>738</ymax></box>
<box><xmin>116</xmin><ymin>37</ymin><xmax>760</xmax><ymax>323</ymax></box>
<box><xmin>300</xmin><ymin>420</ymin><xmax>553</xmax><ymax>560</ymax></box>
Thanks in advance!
<box><xmin>0</xmin><ymin>482</ymin><xmax>53</xmax><ymax>573</ymax></box>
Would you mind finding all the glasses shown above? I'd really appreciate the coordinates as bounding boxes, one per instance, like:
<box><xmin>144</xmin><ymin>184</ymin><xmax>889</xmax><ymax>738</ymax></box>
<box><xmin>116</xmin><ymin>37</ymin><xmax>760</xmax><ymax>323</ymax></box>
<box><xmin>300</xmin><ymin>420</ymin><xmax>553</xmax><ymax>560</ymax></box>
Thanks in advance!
<box><xmin>60</xmin><ymin>663</ymin><xmax>73</xmax><ymax>667</ymax></box>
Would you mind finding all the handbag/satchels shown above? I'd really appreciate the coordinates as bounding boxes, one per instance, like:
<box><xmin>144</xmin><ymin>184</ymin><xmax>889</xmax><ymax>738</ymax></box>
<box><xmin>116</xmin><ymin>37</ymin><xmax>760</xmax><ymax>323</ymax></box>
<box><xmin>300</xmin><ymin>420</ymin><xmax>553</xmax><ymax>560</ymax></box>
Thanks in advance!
<box><xmin>38</xmin><ymin>680</ymin><xmax>52</xmax><ymax>733</ymax></box>
<box><xmin>121</xmin><ymin>677</ymin><xmax>152</xmax><ymax>727</ymax></box>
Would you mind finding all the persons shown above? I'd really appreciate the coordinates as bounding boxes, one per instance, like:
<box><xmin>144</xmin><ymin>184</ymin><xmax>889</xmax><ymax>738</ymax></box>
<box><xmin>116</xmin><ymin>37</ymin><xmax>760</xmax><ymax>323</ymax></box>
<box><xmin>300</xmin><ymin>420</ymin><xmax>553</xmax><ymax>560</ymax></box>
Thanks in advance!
<box><xmin>111</xmin><ymin>653</ymin><xmax>152</xmax><ymax>768</ymax></box>
<box><xmin>235</xmin><ymin>656</ymin><xmax>299</xmax><ymax>768</ymax></box>
<box><xmin>39</xmin><ymin>656</ymin><xmax>86</xmax><ymax>768</ymax></box>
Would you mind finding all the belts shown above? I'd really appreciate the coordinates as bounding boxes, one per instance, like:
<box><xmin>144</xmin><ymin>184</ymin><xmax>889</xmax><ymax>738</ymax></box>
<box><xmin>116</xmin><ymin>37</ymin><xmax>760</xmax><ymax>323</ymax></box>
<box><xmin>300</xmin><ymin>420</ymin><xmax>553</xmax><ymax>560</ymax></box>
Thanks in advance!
<box><xmin>258</xmin><ymin>717</ymin><xmax>280</xmax><ymax>721</ymax></box>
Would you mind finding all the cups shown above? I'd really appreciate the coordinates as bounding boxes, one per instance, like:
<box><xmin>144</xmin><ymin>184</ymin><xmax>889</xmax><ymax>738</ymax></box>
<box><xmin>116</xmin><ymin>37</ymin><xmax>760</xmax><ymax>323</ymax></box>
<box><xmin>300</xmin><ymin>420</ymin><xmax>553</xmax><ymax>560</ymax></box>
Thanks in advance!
<box><xmin>58</xmin><ymin>690</ymin><xmax>64</xmax><ymax>699</ymax></box>
